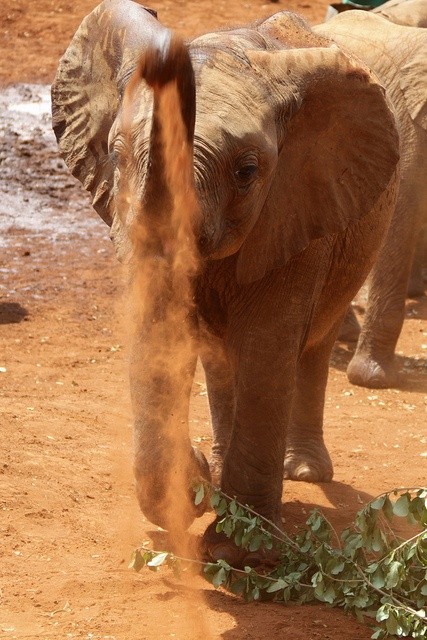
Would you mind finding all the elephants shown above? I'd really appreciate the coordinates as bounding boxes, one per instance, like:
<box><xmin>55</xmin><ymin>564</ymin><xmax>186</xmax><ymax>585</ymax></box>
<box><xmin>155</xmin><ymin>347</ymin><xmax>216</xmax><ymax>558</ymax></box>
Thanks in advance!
<box><xmin>51</xmin><ymin>0</ymin><xmax>402</xmax><ymax>569</ymax></box>
<box><xmin>309</xmin><ymin>0</ymin><xmax>427</xmax><ymax>389</ymax></box>
